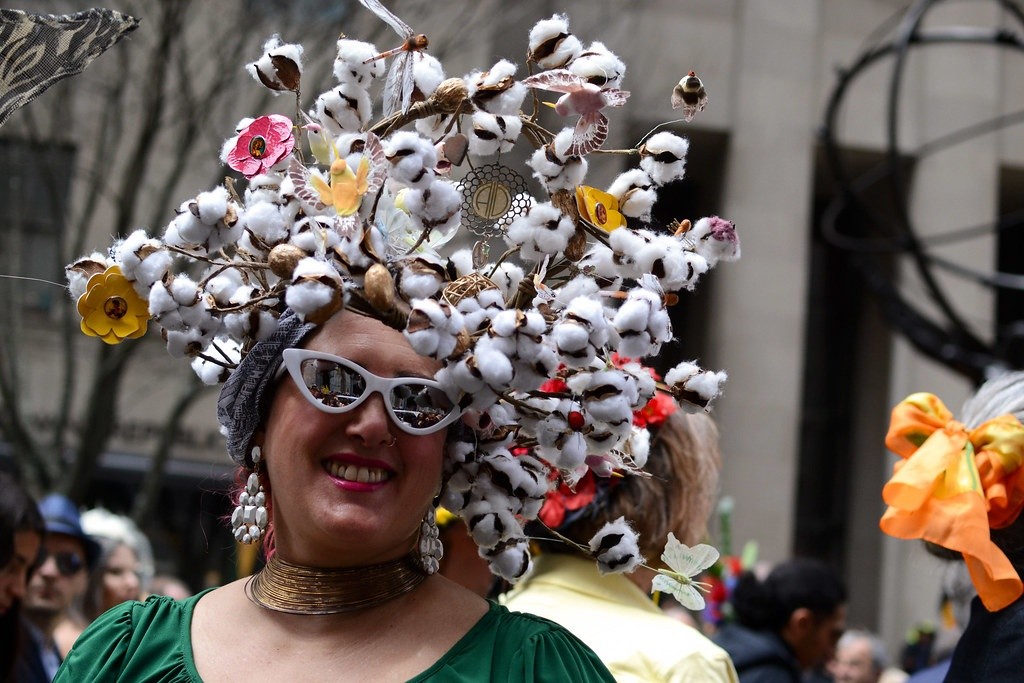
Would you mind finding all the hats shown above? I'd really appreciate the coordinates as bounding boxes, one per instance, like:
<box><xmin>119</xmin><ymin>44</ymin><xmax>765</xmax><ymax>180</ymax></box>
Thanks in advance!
<box><xmin>38</xmin><ymin>493</ymin><xmax>103</xmax><ymax>573</ymax></box>
<box><xmin>79</xmin><ymin>507</ymin><xmax>154</xmax><ymax>591</ymax></box>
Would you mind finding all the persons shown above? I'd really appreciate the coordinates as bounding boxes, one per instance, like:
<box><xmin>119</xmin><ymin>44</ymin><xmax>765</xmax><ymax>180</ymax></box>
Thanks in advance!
<box><xmin>905</xmin><ymin>563</ymin><xmax>977</xmax><ymax>683</ymax></box>
<box><xmin>663</xmin><ymin>557</ymin><xmax>888</xmax><ymax>683</ymax></box>
<box><xmin>307</xmin><ymin>383</ymin><xmax>359</xmax><ymax>407</ymax></box>
<box><xmin>54</xmin><ymin>290</ymin><xmax>616</xmax><ymax>683</ymax></box>
<box><xmin>498</xmin><ymin>406</ymin><xmax>739</xmax><ymax>683</ymax></box>
<box><xmin>392</xmin><ymin>406</ymin><xmax>445</xmax><ymax>429</ymax></box>
<box><xmin>878</xmin><ymin>372</ymin><xmax>1024</xmax><ymax>683</ymax></box>
<box><xmin>0</xmin><ymin>472</ymin><xmax>190</xmax><ymax>683</ymax></box>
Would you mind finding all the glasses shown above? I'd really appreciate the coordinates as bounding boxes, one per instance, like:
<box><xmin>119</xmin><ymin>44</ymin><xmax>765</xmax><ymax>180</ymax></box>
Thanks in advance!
<box><xmin>32</xmin><ymin>548</ymin><xmax>85</xmax><ymax>577</ymax></box>
<box><xmin>275</xmin><ymin>348</ymin><xmax>468</xmax><ymax>436</ymax></box>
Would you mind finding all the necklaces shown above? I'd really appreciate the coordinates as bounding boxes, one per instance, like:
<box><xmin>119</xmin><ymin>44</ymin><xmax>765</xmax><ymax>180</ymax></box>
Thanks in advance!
<box><xmin>244</xmin><ymin>555</ymin><xmax>428</xmax><ymax>614</ymax></box>
